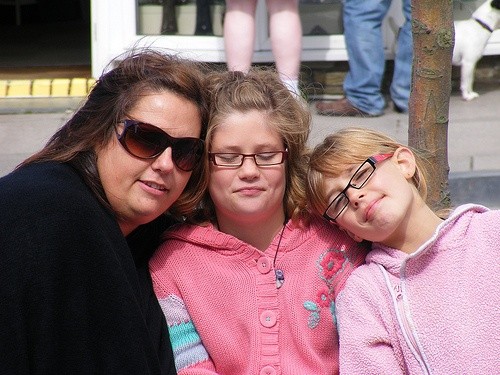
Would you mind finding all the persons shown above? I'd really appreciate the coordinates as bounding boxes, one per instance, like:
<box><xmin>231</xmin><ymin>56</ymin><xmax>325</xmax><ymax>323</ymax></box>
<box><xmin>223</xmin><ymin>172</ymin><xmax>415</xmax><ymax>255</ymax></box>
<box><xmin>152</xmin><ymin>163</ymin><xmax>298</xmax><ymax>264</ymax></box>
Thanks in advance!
<box><xmin>306</xmin><ymin>128</ymin><xmax>500</xmax><ymax>375</ymax></box>
<box><xmin>0</xmin><ymin>35</ymin><xmax>212</xmax><ymax>374</ymax></box>
<box><xmin>148</xmin><ymin>66</ymin><xmax>370</xmax><ymax>375</ymax></box>
<box><xmin>314</xmin><ymin>0</ymin><xmax>413</xmax><ymax>116</ymax></box>
<box><xmin>223</xmin><ymin>0</ymin><xmax>303</xmax><ymax>100</ymax></box>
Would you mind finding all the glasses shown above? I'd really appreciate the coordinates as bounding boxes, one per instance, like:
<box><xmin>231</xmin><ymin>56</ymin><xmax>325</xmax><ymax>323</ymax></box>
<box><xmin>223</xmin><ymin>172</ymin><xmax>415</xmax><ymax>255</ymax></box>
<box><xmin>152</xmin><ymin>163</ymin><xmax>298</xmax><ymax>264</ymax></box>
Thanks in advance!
<box><xmin>323</xmin><ymin>153</ymin><xmax>393</xmax><ymax>223</ymax></box>
<box><xmin>112</xmin><ymin>119</ymin><xmax>206</xmax><ymax>172</ymax></box>
<box><xmin>208</xmin><ymin>147</ymin><xmax>288</xmax><ymax>166</ymax></box>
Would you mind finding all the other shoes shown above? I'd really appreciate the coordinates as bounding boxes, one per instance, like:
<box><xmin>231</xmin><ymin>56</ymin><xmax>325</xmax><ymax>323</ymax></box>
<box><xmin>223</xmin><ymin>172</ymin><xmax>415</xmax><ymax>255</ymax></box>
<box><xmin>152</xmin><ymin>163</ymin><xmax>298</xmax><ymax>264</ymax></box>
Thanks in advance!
<box><xmin>394</xmin><ymin>103</ymin><xmax>403</xmax><ymax>113</ymax></box>
<box><xmin>316</xmin><ymin>97</ymin><xmax>379</xmax><ymax>117</ymax></box>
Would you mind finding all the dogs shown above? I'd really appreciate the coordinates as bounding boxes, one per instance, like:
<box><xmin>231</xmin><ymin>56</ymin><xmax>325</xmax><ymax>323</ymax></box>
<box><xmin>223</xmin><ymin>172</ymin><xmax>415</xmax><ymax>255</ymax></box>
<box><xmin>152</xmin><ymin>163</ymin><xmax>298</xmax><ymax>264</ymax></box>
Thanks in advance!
<box><xmin>454</xmin><ymin>0</ymin><xmax>500</xmax><ymax>102</ymax></box>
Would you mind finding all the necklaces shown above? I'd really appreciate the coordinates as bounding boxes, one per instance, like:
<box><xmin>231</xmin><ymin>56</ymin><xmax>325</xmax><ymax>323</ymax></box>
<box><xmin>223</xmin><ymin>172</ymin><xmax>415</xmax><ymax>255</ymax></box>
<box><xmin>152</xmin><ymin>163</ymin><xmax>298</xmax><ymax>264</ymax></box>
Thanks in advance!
<box><xmin>272</xmin><ymin>224</ymin><xmax>285</xmax><ymax>289</ymax></box>
